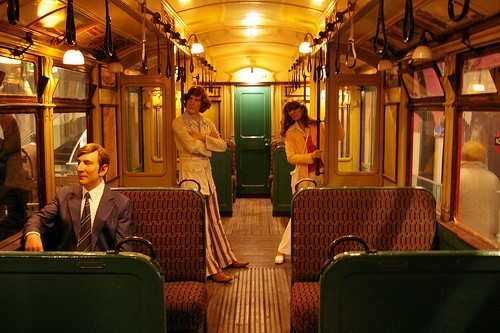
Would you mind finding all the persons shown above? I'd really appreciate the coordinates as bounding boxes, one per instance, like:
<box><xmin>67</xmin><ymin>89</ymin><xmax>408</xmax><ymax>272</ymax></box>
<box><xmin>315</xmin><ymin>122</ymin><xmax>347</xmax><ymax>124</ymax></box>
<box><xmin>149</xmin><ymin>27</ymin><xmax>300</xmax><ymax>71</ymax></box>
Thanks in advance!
<box><xmin>171</xmin><ymin>85</ymin><xmax>250</xmax><ymax>282</ymax></box>
<box><xmin>0</xmin><ymin>70</ymin><xmax>22</xmax><ymax>242</ymax></box>
<box><xmin>413</xmin><ymin>110</ymin><xmax>500</xmax><ymax>245</ymax></box>
<box><xmin>274</xmin><ymin>98</ymin><xmax>326</xmax><ymax>264</ymax></box>
<box><xmin>19</xmin><ymin>143</ymin><xmax>134</xmax><ymax>252</ymax></box>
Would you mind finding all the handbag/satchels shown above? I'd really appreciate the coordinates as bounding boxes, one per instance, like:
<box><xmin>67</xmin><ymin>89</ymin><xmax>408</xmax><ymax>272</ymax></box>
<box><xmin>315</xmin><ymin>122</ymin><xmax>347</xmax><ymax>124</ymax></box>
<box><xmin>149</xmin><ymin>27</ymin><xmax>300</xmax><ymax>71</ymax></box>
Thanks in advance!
<box><xmin>290</xmin><ymin>168</ymin><xmax>299</xmax><ymax>194</ymax></box>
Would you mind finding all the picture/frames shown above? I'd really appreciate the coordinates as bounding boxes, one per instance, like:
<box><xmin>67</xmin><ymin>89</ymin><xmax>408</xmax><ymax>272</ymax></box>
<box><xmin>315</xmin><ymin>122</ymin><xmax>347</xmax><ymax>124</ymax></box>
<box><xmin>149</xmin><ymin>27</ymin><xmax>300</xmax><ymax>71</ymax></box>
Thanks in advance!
<box><xmin>98</xmin><ymin>63</ymin><xmax>118</xmax><ymax>90</ymax></box>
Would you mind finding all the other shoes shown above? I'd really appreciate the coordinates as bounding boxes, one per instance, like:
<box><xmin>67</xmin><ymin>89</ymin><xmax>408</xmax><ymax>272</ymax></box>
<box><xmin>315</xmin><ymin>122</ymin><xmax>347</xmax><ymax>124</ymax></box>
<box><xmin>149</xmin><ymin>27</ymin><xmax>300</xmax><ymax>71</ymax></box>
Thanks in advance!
<box><xmin>275</xmin><ymin>253</ymin><xmax>285</xmax><ymax>264</ymax></box>
<box><xmin>231</xmin><ymin>261</ymin><xmax>249</xmax><ymax>267</ymax></box>
<box><xmin>211</xmin><ymin>272</ymin><xmax>231</xmax><ymax>282</ymax></box>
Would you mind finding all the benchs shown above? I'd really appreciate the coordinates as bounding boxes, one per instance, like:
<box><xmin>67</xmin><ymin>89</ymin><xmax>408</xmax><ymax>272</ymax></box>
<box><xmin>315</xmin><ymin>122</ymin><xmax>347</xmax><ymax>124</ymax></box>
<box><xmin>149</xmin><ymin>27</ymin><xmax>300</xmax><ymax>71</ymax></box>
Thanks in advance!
<box><xmin>108</xmin><ymin>179</ymin><xmax>208</xmax><ymax>333</ymax></box>
<box><xmin>268</xmin><ymin>140</ymin><xmax>500</xmax><ymax>333</ymax></box>
<box><xmin>0</xmin><ymin>237</ymin><xmax>166</xmax><ymax>333</ymax></box>
<box><xmin>210</xmin><ymin>139</ymin><xmax>237</xmax><ymax>217</ymax></box>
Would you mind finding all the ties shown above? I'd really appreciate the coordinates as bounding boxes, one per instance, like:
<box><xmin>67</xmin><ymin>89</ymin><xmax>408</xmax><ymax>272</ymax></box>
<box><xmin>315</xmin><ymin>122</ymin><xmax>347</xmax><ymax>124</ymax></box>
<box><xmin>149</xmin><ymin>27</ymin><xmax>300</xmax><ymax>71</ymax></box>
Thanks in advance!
<box><xmin>77</xmin><ymin>192</ymin><xmax>91</xmax><ymax>252</ymax></box>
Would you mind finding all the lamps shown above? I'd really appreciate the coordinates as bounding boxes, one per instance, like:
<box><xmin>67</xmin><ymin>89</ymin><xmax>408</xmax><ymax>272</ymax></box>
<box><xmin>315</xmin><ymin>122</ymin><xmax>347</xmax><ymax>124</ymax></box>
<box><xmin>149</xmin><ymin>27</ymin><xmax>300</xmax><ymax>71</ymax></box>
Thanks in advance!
<box><xmin>186</xmin><ymin>34</ymin><xmax>204</xmax><ymax>54</ymax></box>
<box><xmin>299</xmin><ymin>33</ymin><xmax>315</xmax><ymax>54</ymax></box>
<box><xmin>412</xmin><ymin>29</ymin><xmax>448</xmax><ymax>60</ymax></box>
<box><xmin>378</xmin><ymin>46</ymin><xmax>403</xmax><ymax>71</ymax></box>
<box><xmin>96</xmin><ymin>49</ymin><xmax>123</xmax><ymax>73</ymax></box>
<box><xmin>51</xmin><ymin>32</ymin><xmax>85</xmax><ymax>65</ymax></box>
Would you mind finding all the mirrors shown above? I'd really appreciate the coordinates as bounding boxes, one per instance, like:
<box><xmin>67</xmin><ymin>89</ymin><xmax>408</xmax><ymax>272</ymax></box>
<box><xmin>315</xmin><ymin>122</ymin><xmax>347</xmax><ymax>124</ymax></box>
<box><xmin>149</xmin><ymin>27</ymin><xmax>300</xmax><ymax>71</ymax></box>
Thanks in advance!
<box><xmin>397</xmin><ymin>60</ymin><xmax>446</xmax><ymax>102</ymax></box>
